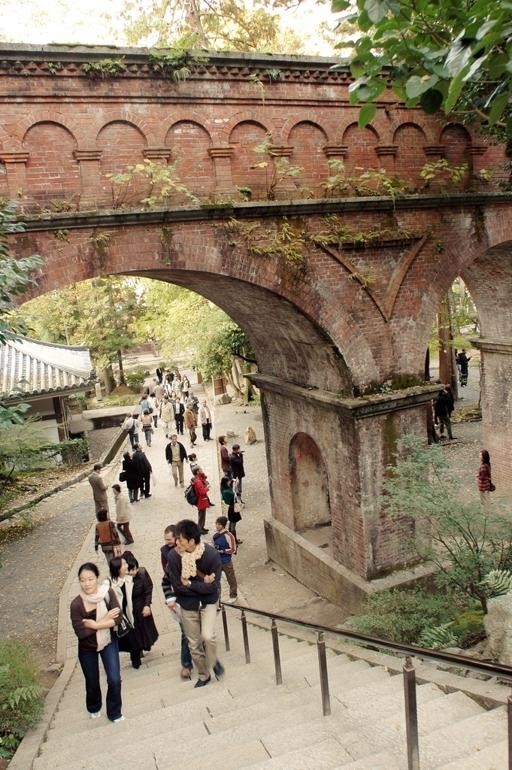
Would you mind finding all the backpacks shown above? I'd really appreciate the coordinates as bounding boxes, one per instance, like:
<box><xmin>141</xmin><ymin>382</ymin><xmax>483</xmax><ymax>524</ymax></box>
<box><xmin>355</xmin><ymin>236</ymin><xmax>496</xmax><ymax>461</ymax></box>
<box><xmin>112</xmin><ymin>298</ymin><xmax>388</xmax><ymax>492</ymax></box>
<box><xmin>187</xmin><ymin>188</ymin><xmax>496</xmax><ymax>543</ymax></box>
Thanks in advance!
<box><xmin>184</xmin><ymin>485</ymin><xmax>197</xmax><ymax>505</ymax></box>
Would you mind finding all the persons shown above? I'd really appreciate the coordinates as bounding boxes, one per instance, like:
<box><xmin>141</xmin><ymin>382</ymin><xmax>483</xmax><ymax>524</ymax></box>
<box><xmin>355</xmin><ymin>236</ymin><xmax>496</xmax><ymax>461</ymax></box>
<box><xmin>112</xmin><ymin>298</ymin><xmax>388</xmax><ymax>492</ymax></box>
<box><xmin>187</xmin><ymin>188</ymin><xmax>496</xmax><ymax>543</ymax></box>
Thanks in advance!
<box><xmin>71</xmin><ymin>510</ymin><xmax>237</xmax><ymax>722</ymax></box>
<box><xmin>453</xmin><ymin>347</ymin><xmax>459</xmax><ymax>384</ymax></box>
<box><xmin>89</xmin><ymin>434</ymin><xmax>245</xmax><ymax>544</ymax></box>
<box><xmin>446</xmin><ymin>384</ymin><xmax>455</xmax><ymax>418</ymax></box>
<box><xmin>458</xmin><ymin>347</ymin><xmax>473</xmax><ymax>388</ymax></box>
<box><xmin>432</xmin><ymin>380</ymin><xmax>444</xmax><ymax>425</ymax></box>
<box><xmin>476</xmin><ymin>450</ymin><xmax>492</xmax><ymax>509</ymax></box>
<box><xmin>436</xmin><ymin>390</ymin><xmax>457</xmax><ymax>440</ymax></box>
<box><xmin>125</xmin><ymin>366</ymin><xmax>214</xmax><ymax>451</ymax></box>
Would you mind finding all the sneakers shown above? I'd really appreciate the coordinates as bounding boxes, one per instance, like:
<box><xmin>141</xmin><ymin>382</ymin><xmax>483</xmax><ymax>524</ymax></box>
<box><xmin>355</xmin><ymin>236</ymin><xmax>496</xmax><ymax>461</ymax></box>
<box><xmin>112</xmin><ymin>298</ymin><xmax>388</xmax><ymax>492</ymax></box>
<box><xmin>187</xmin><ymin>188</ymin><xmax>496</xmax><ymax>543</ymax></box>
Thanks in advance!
<box><xmin>90</xmin><ymin>711</ymin><xmax>101</xmax><ymax>719</ymax></box>
<box><xmin>213</xmin><ymin>661</ymin><xmax>224</xmax><ymax>675</ymax></box>
<box><xmin>114</xmin><ymin>715</ymin><xmax>126</xmax><ymax>723</ymax></box>
<box><xmin>194</xmin><ymin>676</ymin><xmax>211</xmax><ymax>688</ymax></box>
<box><xmin>181</xmin><ymin>667</ymin><xmax>191</xmax><ymax>678</ymax></box>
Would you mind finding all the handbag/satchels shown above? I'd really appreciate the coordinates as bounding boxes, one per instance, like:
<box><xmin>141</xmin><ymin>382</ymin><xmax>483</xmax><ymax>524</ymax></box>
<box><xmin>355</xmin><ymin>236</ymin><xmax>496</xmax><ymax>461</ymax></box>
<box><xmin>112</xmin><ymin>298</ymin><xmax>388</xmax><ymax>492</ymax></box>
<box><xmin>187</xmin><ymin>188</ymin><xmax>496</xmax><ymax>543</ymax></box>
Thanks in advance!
<box><xmin>119</xmin><ymin>471</ymin><xmax>126</xmax><ymax>482</ymax></box>
<box><xmin>114</xmin><ymin>614</ymin><xmax>133</xmax><ymax>638</ymax></box>
<box><xmin>234</xmin><ymin>501</ymin><xmax>245</xmax><ymax>513</ymax></box>
<box><xmin>112</xmin><ymin>544</ymin><xmax>124</xmax><ymax>558</ymax></box>
